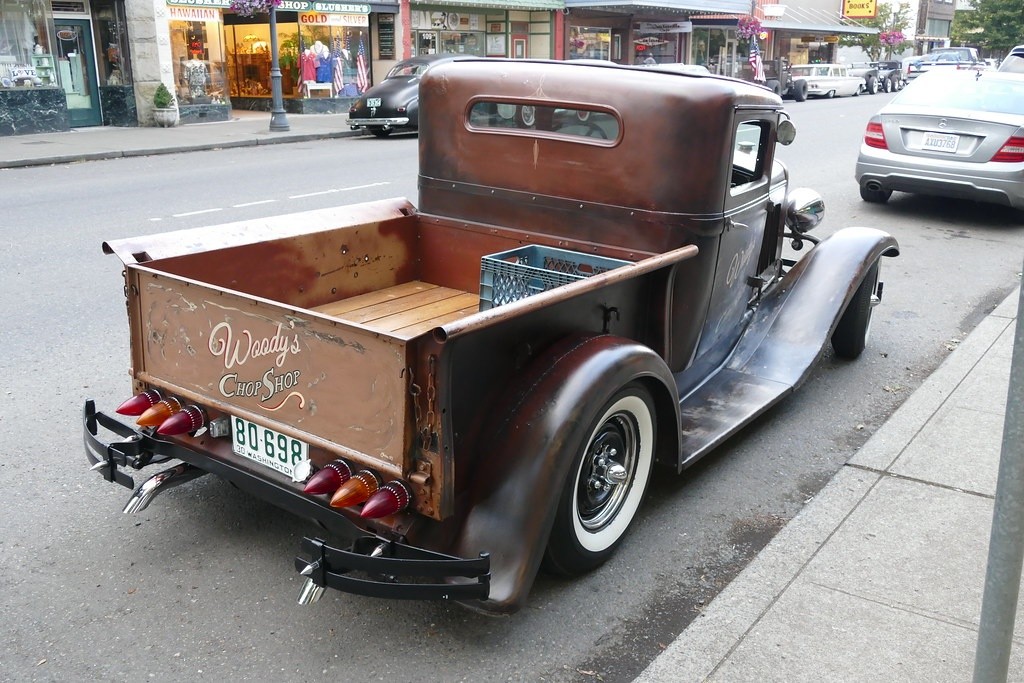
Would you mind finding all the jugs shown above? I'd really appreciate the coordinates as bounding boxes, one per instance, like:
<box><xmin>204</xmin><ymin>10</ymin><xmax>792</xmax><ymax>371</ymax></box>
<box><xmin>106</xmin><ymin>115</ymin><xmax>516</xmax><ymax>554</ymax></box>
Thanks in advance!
<box><xmin>33</xmin><ymin>44</ymin><xmax>44</xmax><ymax>55</ymax></box>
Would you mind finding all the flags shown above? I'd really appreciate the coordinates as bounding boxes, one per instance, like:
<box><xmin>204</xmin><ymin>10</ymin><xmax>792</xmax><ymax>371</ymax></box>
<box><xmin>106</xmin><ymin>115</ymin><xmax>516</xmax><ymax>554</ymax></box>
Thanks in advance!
<box><xmin>346</xmin><ymin>34</ymin><xmax>350</xmax><ymax>53</ymax></box>
<box><xmin>749</xmin><ymin>38</ymin><xmax>766</xmax><ymax>82</ymax></box>
<box><xmin>357</xmin><ymin>36</ymin><xmax>368</xmax><ymax>93</ymax></box>
<box><xmin>332</xmin><ymin>34</ymin><xmax>344</xmax><ymax>92</ymax></box>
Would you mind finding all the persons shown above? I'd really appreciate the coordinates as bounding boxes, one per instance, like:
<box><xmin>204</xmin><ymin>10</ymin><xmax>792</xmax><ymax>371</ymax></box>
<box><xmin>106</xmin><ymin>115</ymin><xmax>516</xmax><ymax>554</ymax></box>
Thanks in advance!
<box><xmin>708</xmin><ymin>59</ymin><xmax>716</xmax><ymax>74</ymax></box>
<box><xmin>637</xmin><ymin>52</ymin><xmax>644</xmax><ymax>64</ymax></box>
<box><xmin>643</xmin><ymin>52</ymin><xmax>656</xmax><ymax>64</ymax></box>
<box><xmin>297</xmin><ymin>40</ymin><xmax>334</xmax><ymax>93</ymax></box>
<box><xmin>184</xmin><ymin>58</ymin><xmax>209</xmax><ymax>97</ymax></box>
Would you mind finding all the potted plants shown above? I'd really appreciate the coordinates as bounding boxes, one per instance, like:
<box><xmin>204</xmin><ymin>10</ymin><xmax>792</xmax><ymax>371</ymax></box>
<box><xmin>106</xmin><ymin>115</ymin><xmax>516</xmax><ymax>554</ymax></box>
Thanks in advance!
<box><xmin>152</xmin><ymin>83</ymin><xmax>176</xmax><ymax>129</ymax></box>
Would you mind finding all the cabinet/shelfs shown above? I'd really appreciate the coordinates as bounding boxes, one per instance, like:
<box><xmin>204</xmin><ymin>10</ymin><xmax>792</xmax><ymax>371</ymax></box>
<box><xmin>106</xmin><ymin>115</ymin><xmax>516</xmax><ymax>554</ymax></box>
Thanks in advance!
<box><xmin>32</xmin><ymin>55</ymin><xmax>58</xmax><ymax>86</ymax></box>
<box><xmin>231</xmin><ymin>54</ymin><xmax>268</xmax><ymax>89</ymax></box>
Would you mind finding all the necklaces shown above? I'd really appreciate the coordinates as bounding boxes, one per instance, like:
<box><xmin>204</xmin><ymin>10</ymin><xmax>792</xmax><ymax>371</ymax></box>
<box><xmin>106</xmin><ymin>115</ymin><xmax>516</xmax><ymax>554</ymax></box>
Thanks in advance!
<box><xmin>314</xmin><ymin>45</ymin><xmax>323</xmax><ymax>54</ymax></box>
<box><xmin>302</xmin><ymin>52</ymin><xmax>313</xmax><ymax>61</ymax></box>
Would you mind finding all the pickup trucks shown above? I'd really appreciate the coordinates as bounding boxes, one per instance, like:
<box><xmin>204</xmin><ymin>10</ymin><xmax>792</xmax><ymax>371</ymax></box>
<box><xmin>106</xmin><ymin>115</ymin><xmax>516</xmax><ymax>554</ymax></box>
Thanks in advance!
<box><xmin>80</xmin><ymin>53</ymin><xmax>899</xmax><ymax>618</ymax></box>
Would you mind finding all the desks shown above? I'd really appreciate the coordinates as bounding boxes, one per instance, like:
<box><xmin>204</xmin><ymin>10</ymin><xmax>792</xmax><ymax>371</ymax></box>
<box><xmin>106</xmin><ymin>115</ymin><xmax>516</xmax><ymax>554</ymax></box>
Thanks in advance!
<box><xmin>306</xmin><ymin>83</ymin><xmax>332</xmax><ymax>97</ymax></box>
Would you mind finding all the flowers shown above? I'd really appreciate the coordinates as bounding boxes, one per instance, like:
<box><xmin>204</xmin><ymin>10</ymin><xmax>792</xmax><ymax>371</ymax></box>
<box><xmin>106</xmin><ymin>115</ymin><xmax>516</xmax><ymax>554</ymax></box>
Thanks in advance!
<box><xmin>735</xmin><ymin>16</ymin><xmax>764</xmax><ymax>40</ymax></box>
<box><xmin>879</xmin><ymin>32</ymin><xmax>906</xmax><ymax>47</ymax></box>
<box><xmin>230</xmin><ymin>0</ymin><xmax>282</xmax><ymax>19</ymax></box>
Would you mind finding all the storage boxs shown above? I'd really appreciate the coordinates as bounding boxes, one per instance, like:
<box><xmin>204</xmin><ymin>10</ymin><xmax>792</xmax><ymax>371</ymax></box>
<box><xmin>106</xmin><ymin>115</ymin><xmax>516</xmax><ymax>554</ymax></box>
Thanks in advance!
<box><xmin>479</xmin><ymin>245</ymin><xmax>634</xmax><ymax>313</ymax></box>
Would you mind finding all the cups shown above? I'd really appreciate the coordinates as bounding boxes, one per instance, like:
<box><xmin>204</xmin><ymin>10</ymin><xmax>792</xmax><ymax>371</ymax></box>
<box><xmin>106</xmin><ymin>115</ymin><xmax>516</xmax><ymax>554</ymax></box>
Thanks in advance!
<box><xmin>39</xmin><ymin>58</ymin><xmax>49</xmax><ymax>66</ymax></box>
<box><xmin>24</xmin><ymin>80</ymin><xmax>30</xmax><ymax>88</ymax></box>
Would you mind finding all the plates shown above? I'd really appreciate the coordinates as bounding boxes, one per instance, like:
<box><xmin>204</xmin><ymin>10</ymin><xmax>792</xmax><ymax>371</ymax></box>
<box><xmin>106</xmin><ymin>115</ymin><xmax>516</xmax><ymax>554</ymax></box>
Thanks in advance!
<box><xmin>57</xmin><ymin>30</ymin><xmax>76</xmax><ymax>40</ymax></box>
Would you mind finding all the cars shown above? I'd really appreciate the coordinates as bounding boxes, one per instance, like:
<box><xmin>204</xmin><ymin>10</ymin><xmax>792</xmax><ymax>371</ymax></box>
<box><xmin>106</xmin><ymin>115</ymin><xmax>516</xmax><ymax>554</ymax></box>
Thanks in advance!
<box><xmin>345</xmin><ymin>51</ymin><xmax>535</xmax><ymax>137</ymax></box>
<box><xmin>854</xmin><ymin>66</ymin><xmax>1024</xmax><ymax>213</ymax></box>
<box><xmin>740</xmin><ymin>45</ymin><xmax>1024</xmax><ymax>103</ymax></box>
<box><xmin>552</xmin><ymin>57</ymin><xmax>712</xmax><ymax>125</ymax></box>
